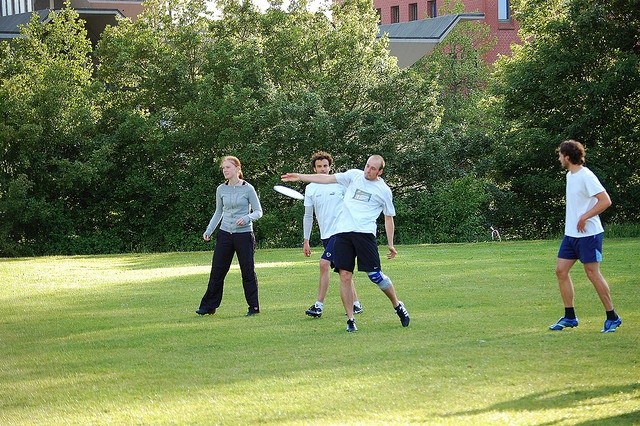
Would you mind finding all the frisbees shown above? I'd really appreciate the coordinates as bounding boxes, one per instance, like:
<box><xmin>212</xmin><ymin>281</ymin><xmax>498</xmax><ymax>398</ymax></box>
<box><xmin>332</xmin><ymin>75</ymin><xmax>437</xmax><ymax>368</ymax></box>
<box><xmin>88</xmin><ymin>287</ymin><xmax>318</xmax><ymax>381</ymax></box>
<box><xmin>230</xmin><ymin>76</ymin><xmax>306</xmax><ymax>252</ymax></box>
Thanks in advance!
<box><xmin>273</xmin><ymin>186</ymin><xmax>304</xmax><ymax>199</ymax></box>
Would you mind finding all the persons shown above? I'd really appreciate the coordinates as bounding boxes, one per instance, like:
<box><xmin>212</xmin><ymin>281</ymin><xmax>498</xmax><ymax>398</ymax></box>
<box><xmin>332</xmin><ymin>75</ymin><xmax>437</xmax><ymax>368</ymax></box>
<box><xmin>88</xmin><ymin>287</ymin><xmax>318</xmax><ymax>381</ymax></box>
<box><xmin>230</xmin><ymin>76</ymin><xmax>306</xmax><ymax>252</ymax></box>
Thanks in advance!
<box><xmin>303</xmin><ymin>151</ymin><xmax>362</xmax><ymax>316</ymax></box>
<box><xmin>547</xmin><ymin>140</ymin><xmax>622</xmax><ymax>333</ymax></box>
<box><xmin>279</xmin><ymin>146</ymin><xmax>411</xmax><ymax>334</ymax></box>
<box><xmin>195</xmin><ymin>154</ymin><xmax>264</xmax><ymax>316</ymax></box>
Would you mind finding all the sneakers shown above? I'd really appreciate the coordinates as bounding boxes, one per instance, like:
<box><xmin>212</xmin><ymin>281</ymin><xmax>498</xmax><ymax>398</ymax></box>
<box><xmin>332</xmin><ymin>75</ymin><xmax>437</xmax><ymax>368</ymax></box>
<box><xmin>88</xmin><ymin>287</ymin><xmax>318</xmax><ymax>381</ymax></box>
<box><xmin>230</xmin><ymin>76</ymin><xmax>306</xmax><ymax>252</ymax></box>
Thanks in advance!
<box><xmin>344</xmin><ymin>305</ymin><xmax>362</xmax><ymax>315</ymax></box>
<box><xmin>347</xmin><ymin>318</ymin><xmax>358</xmax><ymax>333</ymax></box>
<box><xmin>196</xmin><ymin>309</ymin><xmax>216</xmax><ymax>315</ymax></box>
<box><xmin>394</xmin><ymin>301</ymin><xmax>410</xmax><ymax>327</ymax></box>
<box><xmin>601</xmin><ymin>316</ymin><xmax>621</xmax><ymax>333</ymax></box>
<box><xmin>549</xmin><ymin>316</ymin><xmax>579</xmax><ymax>330</ymax></box>
<box><xmin>305</xmin><ymin>305</ymin><xmax>322</xmax><ymax>318</ymax></box>
<box><xmin>245</xmin><ymin>307</ymin><xmax>259</xmax><ymax>316</ymax></box>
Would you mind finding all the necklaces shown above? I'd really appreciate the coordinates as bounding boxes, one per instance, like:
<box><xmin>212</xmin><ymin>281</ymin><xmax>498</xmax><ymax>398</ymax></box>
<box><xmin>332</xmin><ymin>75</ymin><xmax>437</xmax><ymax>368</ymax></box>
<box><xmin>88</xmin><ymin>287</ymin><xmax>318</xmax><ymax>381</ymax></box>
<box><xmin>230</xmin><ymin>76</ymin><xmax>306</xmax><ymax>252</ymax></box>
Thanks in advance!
<box><xmin>228</xmin><ymin>181</ymin><xmax>238</xmax><ymax>188</ymax></box>
